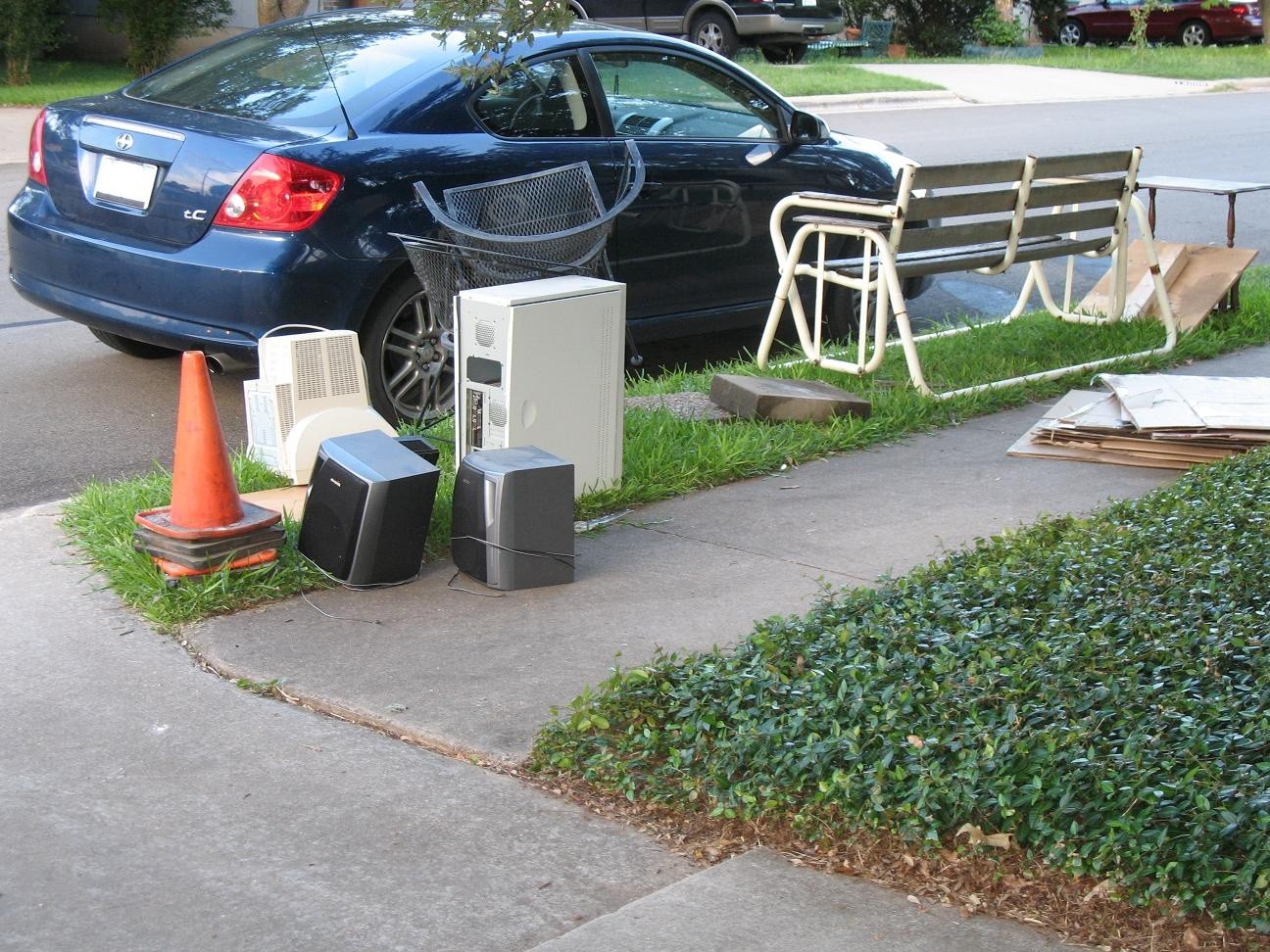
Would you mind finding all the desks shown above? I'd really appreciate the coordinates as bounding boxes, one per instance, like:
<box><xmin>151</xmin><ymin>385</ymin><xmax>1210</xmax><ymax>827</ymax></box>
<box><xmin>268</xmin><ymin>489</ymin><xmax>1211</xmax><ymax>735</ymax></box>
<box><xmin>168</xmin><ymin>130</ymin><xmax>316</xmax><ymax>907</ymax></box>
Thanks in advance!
<box><xmin>1143</xmin><ymin>175</ymin><xmax>1269</xmax><ymax>310</ymax></box>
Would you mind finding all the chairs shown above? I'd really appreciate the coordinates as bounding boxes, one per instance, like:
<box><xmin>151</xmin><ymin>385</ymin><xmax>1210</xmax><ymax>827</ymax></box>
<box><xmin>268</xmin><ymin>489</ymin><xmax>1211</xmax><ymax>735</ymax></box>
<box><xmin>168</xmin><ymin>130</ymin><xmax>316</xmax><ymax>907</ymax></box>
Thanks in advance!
<box><xmin>388</xmin><ymin>144</ymin><xmax>651</xmax><ymax>444</ymax></box>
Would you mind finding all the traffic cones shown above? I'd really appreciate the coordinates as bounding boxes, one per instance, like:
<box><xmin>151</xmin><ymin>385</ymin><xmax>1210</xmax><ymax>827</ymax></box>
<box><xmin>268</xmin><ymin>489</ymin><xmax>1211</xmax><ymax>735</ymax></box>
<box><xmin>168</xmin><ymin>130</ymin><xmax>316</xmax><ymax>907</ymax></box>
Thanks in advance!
<box><xmin>131</xmin><ymin>351</ymin><xmax>289</xmax><ymax>580</ymax></box>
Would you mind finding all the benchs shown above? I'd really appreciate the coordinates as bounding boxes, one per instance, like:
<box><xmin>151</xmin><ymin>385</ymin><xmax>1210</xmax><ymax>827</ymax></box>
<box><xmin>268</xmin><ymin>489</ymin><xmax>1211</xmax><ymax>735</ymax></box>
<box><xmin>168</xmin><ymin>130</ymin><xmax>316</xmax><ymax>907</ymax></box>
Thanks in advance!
<box><xmin>753</xmin><ymin>146</ymin><xmax>1176</xmax><ymax>400</ymax></box>
<box><xmin>601</xmin><ymin>172</ymin><xmax>757</xmax><ymax>270</ymax></box>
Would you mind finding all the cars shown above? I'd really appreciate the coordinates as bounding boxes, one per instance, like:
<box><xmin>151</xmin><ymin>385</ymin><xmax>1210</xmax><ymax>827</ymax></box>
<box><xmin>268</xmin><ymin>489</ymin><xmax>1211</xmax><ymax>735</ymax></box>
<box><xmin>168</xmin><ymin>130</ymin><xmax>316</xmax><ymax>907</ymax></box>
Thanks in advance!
<box><xmin>1039</xmin><ymin>0</ymin><xmax>1264</xmax><ymax>48</ymax></box>
<box><xmin>5</xmin><ymin>6</ymin><xmax>940</xmax><ymax>435</ymax></box>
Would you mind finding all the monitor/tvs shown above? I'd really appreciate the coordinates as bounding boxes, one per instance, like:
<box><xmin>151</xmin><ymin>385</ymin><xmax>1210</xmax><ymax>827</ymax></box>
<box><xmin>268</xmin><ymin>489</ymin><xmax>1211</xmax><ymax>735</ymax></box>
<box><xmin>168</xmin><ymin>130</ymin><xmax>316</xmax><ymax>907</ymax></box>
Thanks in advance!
<box><xmin>242</xmin><ymin>330</ymin><xmax>400</xmax><ymax>485</ymax></box>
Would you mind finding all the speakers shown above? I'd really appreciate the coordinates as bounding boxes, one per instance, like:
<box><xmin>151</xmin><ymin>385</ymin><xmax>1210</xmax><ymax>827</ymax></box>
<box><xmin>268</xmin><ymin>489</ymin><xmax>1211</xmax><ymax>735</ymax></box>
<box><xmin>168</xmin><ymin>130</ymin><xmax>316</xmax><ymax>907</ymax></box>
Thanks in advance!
<box><xmin>451</xmin><ymin>444</ymin><xmax>576</xmax><ymax>591</ymax></box>
<box><xmin>293</xmin><ymin>429</ymin><xmax>442</xmax><ymax>588</ymax></box>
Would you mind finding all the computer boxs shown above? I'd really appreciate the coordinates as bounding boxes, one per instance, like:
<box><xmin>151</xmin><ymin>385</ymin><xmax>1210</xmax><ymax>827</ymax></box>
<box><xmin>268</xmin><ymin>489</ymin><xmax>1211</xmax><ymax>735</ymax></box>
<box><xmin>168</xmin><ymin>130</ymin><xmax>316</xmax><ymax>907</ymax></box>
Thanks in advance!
<box><xmin>454</xmin><ymin>274</ymin><xmax>628</xmax><ymax>499</ymax></box>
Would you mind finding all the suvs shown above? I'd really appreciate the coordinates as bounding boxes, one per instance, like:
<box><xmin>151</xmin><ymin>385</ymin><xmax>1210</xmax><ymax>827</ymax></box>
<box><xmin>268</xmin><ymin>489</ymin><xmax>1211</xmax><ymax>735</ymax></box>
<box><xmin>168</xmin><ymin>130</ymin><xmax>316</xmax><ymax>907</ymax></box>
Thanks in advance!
<box><xmin>543</xmin><ymin>0</ymin><xmax>848</xmax><ymax>58</ymax></box>
